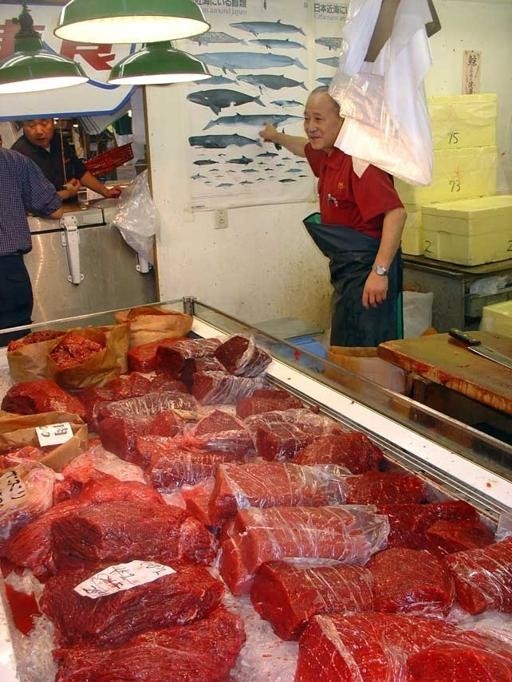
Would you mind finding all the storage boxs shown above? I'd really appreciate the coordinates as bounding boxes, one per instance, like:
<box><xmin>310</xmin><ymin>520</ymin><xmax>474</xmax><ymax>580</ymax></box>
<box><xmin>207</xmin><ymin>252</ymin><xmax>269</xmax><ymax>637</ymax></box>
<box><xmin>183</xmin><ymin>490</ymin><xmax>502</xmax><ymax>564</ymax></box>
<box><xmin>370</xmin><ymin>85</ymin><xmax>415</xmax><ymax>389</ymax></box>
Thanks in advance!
<box><xmin>477</xmin><ymin>298</ymin><xmax>512</xmax><ymax>337</ymax></box>
<box><xmin>393</xmin><ymin>92</ymin><xmax>511</xmax><ymax>268</ymax></box>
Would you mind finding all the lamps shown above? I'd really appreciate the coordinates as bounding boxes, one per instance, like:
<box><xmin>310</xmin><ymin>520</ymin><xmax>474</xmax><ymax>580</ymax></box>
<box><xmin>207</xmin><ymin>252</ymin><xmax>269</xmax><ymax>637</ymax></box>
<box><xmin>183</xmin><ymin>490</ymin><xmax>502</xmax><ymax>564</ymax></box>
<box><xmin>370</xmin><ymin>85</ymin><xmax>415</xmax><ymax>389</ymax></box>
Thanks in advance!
<box><xmin>105</xmin><ymin>41</ymin><xmax>214</xmax><ymax>86</ymax></box>
<box><xmin>53</xmin><ymin>1</ymin><xmax>212</xmax><ymax>45</ymax></box>
<box><xmin>0</xmin><ymin>0</ymin><xmax>89</xmax><ymax>95</ymax></box>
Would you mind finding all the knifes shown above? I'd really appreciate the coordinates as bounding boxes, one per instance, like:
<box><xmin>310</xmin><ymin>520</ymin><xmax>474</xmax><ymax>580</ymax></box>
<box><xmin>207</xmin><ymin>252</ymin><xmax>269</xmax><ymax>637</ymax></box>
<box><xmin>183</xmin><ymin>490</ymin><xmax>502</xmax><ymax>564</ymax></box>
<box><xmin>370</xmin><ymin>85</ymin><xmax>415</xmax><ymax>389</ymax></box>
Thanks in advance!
<box><xmin>447</xmin><ymin>327</ymin><xmax>512</xmax><ymax>372</ymax></box>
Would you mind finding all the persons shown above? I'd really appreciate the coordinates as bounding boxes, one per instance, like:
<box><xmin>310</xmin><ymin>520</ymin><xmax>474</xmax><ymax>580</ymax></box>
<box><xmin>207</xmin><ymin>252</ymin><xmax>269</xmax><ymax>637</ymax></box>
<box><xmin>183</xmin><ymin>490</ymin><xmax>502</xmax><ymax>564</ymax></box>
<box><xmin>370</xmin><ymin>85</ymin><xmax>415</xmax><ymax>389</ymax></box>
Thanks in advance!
<box><xmin>1</xmin><ymin>135</ymin><xmax>64</xmax><ymax>348</ymax></box>
<box><xmin>9</xmin><ymin>117</ymin><xmax>122</xmax><ymax>199</ymax></box>
<box><xmin>258</xmin><ymin>85</ymin><xmax>406</xmax><ymax>347</ymax></box>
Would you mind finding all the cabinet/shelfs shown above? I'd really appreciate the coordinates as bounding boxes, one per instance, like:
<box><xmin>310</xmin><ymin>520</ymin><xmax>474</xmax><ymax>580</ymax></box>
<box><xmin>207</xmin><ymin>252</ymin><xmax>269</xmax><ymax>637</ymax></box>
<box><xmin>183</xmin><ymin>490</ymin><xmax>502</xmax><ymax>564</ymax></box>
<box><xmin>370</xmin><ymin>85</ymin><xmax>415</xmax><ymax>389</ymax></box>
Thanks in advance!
<box><xmin>400</xmin><ymin>254</ymin><xmax>511</xmax><ymax>337</ymax></box>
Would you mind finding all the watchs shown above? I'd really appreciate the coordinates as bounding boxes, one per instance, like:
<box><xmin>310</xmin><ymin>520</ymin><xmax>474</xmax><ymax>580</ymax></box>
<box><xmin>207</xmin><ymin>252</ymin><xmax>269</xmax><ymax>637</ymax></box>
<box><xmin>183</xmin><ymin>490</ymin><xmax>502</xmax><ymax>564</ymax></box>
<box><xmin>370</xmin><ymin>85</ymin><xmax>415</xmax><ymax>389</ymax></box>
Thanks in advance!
<box><xmin>371</xmin><ymin>263</ymin><xmax>389</xmax><ymax>276</ymax></box>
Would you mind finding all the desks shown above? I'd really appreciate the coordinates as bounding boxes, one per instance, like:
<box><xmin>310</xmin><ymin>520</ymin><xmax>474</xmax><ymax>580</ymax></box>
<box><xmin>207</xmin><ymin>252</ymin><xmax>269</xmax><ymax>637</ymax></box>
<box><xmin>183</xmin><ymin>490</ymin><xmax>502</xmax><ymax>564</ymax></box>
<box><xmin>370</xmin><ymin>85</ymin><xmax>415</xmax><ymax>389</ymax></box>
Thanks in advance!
<box><xmin>375</xmin><ymin>331</ymin><xmax>511</xmax><ymax>444</ymax></box>
